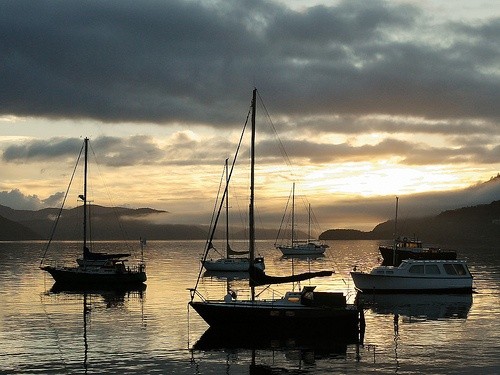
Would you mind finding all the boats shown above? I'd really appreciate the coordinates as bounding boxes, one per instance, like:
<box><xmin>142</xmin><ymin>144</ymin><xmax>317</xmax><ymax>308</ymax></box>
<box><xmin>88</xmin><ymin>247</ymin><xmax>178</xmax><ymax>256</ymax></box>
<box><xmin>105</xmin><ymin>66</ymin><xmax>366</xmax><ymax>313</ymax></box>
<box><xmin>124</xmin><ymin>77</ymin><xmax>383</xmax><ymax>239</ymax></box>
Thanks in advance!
<box><xmin>347</xmin><ymin>292</ymin><xmax>476</xmax><ymax>323</ymax></box>
<box><xmin>380</xmin><ymin>196</ymin><xmax>459</xmax><ymax>261</ymax></box>
<box><xmin>289</xmin><ymin>238</ymin><xmax>323</xmax><ymax>247</ymax></box>
<box><xmin>349</xmin><ymin>259</ymin><xmax>476</xmax><ymax>291</ymax></box>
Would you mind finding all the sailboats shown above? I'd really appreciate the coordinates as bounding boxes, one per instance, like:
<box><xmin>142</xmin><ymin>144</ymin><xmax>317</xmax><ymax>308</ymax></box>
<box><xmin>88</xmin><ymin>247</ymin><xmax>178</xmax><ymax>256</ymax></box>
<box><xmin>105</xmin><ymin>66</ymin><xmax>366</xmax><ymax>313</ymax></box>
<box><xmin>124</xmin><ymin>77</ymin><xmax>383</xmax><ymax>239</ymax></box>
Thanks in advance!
<box><xmin>200</xmin><ymin>159</ymin><xmax>265</xmax><ymax>270</ymax></box>
<box><xmin>39</xmin><ymin>283</ymin><xmax>147</xmax><ymax>374</ymax></box>
<box><xmin>199</xmin><ymin>270</ymin><xmax>266</xmax><ymax>299</ymax></box>
<box><xmin>275</xmin><ymin>253</ymin><xmax>325</xmax><ymax>294</ymax></box>
<box><xmin>188</xmin><ymin>326</ymin><xmax>377</xmax><ymax>375</ymax></box>
<box><xmin>274</xmin><ymin>180</ymin><xmax>328</xmax><ymax>253</ymax></box>
<box><xmin>188</xmin><ymin>86</ymin><xmax>378</xmax><ymax>326</ymax></box>
<box><xmin>38</xmin><ymin>136</ymin><xmax>147</xmax><ymax>283</ymax></box>
